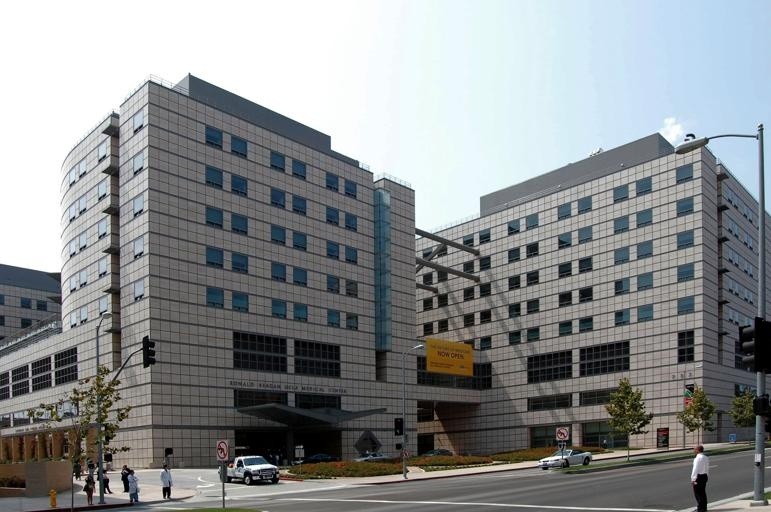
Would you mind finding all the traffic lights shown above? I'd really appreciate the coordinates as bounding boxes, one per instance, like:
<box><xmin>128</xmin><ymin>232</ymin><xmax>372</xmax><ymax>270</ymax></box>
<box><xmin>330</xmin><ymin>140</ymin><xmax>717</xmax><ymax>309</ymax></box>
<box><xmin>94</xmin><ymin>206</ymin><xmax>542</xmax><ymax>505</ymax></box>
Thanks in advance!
<box><xmin>394</xmin><ymin>418</ymin><xmax>404</xmax><ymax>436</ymax></box>
<box><xmin>753</xmin><ymin>394</ymin><xmax>770</xmax><ymax>416</ymax></box>
<box><xmin>142</xmin><ymin>335</ymin><xmax>157</xmax><ymax>368</ymax></box>
<box><xmin>742</xmin><ymin>316</ymin><xmax>762</xmax><ymax>368</ymax></box>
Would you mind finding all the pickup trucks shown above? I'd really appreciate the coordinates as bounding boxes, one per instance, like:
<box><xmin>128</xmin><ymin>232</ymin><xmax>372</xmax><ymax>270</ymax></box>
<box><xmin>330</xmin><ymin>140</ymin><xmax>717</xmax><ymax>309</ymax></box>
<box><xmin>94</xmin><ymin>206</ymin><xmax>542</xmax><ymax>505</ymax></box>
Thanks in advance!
<box><xmin>217</xmin><ymin>455</ymin><xmax>280</xmax><ymax>484</ymax></box>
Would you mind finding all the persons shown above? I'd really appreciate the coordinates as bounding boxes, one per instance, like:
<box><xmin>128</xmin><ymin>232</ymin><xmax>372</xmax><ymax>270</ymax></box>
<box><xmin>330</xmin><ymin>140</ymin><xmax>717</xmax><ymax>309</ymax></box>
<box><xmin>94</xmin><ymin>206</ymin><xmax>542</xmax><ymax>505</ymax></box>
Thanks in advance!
<box><xmin>73</xmin><ymin>457</ymin><xmax>139</xmax><ymax>505</ymax></box>
<box><xmin>160</xmin><ymin>464</ymin><xmax>172</xmax><ymax>499</ymax></box>
<box><xmin>690</xmin><ymin>445</ymin><xmax>710</xmax><ymax>512</ymax></box>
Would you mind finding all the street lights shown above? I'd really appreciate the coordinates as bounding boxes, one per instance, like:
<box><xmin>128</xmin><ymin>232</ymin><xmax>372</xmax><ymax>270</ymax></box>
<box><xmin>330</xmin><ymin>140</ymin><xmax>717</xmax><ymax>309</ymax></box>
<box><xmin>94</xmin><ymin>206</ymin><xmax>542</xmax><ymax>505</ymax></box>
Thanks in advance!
<box><xmin>400</xmin><ymin>345</ymin><xmax>424</xmax><ymax>479</ymax></box>
<box><xmin>673</xmin><ymin>123</ymin><xmax>768</xmax><ymax>504</ymax></box>
<box><xmin>96</xmin><ymin>311</ymin><xmax>114</xmax><ymax>502</ymax></box>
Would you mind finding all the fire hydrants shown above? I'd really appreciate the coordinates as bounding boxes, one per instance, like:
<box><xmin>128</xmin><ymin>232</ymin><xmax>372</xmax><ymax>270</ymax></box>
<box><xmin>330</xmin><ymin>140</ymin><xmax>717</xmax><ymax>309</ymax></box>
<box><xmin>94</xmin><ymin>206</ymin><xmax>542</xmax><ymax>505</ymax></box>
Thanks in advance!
<box><xmin>48</xmin><ymin>489</ymin><xmax>58</xmax><ymax>508</ymax></box>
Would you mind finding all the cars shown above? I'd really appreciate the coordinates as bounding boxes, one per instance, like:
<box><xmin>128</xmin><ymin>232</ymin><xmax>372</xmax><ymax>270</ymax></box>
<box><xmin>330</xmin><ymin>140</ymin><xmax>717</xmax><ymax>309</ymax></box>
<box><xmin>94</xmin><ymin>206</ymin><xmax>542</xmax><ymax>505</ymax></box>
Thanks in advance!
<box><xmin>351</xmin><ymin>452</ymin><xmax>393</xmax><ymax>463</ymax></box>
<box><xmin>422</xmin><ymin>448</ymin><xmax>454</xmax><ymax>457</ymax></box>
<box><xmin>537</xmin><ymin>448</ymin><xmax>591</xmax><ymax>470</ymax></box>
<box><xmin>305</xmin><ymin>454</ymin><xmax>342</xmax><ymax>463</ymax></box>
